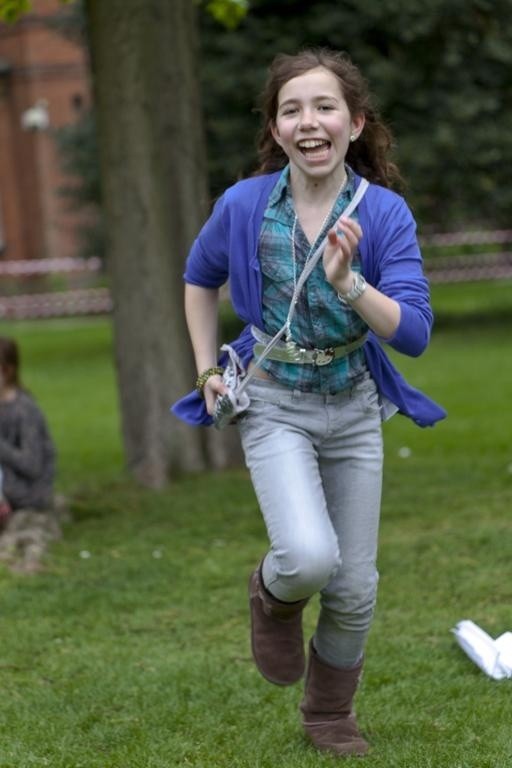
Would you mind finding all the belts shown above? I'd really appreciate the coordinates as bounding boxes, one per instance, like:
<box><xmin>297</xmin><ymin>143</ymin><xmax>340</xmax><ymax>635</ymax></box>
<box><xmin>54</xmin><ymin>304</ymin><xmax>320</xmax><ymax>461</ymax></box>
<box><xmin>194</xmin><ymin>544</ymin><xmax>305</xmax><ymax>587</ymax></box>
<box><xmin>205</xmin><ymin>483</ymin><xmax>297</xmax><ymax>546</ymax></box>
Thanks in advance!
<box><xmin>250</xmin><ymin>324</ymin><xmax>371</xmax><ymax>366</ymax></box>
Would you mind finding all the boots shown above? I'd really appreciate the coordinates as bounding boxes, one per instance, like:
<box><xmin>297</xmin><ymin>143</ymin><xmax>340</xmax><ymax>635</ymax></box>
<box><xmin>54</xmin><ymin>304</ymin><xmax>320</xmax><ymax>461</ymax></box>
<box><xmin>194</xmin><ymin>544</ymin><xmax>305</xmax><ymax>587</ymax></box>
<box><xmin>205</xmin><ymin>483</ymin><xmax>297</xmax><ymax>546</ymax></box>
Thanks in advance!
<box><xmin>247</xmin><ymin>553</ymin><xmax>310</xmax><ymax>687</ymax></box>
<box><xmin>299</xmin><ymin>636</ymin><xmax>370</xmax><ymax>759</ymax></box>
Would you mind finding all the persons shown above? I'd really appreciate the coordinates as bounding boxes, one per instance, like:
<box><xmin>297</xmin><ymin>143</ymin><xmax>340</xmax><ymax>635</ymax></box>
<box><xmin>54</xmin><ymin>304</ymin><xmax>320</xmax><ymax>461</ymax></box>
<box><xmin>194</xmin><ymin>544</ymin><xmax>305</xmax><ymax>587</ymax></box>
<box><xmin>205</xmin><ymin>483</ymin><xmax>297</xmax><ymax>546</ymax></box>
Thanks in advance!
<box><xmin>0</xmin><ymin>333</ymin><xmax>56</xmax><ymax>525</ymax></box>
<box><xmin>172</xmin><ymin>48</ymin><xmax>434</xmax><ymax>754</ymax></box>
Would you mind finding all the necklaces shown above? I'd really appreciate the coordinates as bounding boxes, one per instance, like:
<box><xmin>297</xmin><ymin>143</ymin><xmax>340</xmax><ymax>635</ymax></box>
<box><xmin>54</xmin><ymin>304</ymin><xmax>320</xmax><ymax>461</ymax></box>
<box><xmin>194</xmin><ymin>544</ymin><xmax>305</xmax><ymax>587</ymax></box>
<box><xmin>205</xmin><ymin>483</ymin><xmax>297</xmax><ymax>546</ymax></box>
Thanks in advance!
<box><xmin>284</xmin><ymin>175</ymin><xmax>348</xmax><ymax>352</ymax></box>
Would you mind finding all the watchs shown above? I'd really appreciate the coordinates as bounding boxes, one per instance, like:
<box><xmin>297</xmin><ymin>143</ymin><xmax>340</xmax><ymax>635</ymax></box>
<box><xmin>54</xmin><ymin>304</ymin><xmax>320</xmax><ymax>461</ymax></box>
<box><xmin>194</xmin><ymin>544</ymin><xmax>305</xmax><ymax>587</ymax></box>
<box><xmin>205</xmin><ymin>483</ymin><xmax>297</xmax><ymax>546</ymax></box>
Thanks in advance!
<box><xmin>338</xmin><ymin>271</ymin><xmax>367</xmax><ymax>304</ymax></box>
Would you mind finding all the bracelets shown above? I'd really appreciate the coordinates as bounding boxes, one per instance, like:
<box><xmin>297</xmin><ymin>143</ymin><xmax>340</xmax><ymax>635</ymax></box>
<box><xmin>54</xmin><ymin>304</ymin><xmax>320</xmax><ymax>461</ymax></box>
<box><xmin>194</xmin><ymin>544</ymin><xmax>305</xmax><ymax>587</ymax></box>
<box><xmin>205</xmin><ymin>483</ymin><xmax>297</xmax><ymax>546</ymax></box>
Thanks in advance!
<box><xmin>195</xmin><ymin>367</ymin><xmax>224</xmax><ymax>394</ymax></box>
